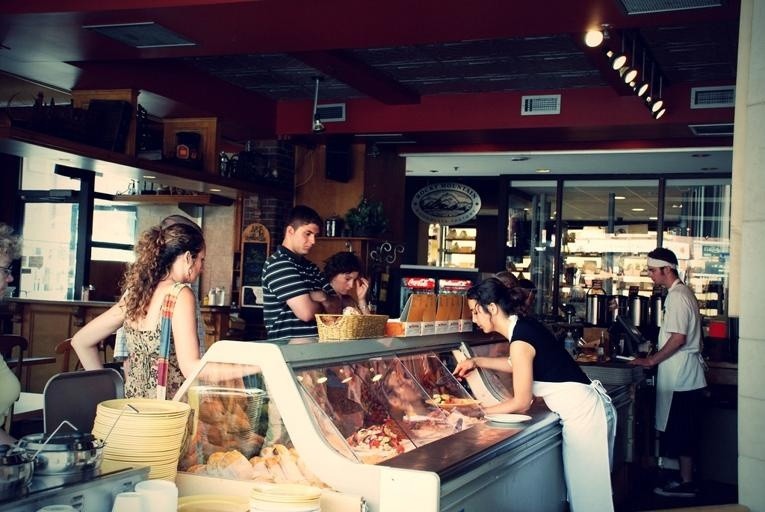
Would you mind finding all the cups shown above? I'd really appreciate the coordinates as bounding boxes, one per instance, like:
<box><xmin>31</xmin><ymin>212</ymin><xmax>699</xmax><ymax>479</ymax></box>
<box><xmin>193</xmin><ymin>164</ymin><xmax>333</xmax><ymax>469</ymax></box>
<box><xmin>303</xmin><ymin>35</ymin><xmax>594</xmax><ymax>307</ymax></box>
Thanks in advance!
<box><xmin>35</xmin><ymin>477</ymin><xmax>179</xmax><ymax>512</ymax></box>
<box><xmin>203</xmin><ymin>285</ymin><xmax>226</xmax><ymax>307</ymax></box>
<box><xmin>79</xmin><ymin>283</ymin><xmax>97</xmax><ymax>302</ymax></box>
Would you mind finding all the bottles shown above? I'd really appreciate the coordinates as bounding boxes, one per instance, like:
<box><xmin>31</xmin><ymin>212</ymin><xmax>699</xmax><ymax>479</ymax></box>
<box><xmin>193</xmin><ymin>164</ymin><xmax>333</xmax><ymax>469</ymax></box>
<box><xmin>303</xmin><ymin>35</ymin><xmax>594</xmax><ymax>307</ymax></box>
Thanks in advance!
<box><xmin>562</xmin><ymin>330</ymin><xmax>574</xmax><ymax>355</ymax></box>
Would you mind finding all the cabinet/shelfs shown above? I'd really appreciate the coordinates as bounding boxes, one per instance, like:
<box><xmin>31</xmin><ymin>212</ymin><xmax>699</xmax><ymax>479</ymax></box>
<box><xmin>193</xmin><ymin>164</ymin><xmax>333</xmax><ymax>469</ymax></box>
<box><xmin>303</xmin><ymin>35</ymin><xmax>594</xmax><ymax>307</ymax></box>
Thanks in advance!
<box><xmin>305</xmin><ymin>236</ymin><xmax>381</xmax><ymax>275</ymax></box>
<box><xmin>427</xmin><ymin>225</ymin><xmax>477</xmax><ymax>270</ymax></box>
<box><xmin>562</xmin><ymin>240</ymin><xmax>690</xmax><ymax>305</ymax></box>
<box><xmin>18</xmin><ymin>303</ymin><xmax>114</xmax><ymax>393</ymax></box>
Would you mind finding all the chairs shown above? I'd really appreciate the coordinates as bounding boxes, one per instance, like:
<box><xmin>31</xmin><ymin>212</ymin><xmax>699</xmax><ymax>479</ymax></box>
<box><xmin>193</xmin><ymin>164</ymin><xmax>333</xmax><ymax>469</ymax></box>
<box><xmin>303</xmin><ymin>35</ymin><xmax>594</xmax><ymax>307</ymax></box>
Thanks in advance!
<box><xmin>104</xmin><ymin>335</ymin><xmax>121</xmax><ymax>368</ymax></box>
<box><xmin>55</xmin><ymin>338</ymin><xmax>83</xmax><ymax>373</ymax></box>
<box><xmin>0</xmin><ymin>333</ymin><xmax>28</xmax><ymax>381</ymax></box>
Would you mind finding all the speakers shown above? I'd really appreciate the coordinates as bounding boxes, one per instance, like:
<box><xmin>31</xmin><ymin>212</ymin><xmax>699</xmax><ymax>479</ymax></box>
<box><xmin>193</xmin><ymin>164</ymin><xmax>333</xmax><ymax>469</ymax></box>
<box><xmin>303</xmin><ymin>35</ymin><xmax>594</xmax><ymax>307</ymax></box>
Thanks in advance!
<box><xmin>326</xmin><ymin>135</ymin><xmax>353</xmax><ymax>182</ymax></box>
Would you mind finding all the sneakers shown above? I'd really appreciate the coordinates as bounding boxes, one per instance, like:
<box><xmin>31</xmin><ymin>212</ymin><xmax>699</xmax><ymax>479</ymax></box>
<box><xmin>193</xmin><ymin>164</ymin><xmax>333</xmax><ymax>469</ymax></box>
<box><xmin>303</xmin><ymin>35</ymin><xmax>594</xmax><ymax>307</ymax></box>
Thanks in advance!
<box><xmin>653</xmin><ymin>482</ymin><xmax>697</xmax><ymax>497</ymax></box>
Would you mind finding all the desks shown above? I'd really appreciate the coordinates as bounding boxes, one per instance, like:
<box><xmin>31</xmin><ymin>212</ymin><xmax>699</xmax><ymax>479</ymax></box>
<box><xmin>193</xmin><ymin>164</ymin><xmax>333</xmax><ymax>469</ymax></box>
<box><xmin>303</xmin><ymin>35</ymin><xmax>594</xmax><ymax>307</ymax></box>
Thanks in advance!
<box><xmin>13</xmin><ymin>391</ymin><xmax>45</xmax><ymax>421</ymax></box>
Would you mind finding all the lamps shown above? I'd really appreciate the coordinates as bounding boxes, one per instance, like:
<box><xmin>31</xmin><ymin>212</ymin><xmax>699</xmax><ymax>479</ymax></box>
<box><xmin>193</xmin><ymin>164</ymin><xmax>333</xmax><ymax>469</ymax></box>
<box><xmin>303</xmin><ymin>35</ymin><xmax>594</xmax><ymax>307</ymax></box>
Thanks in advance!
<box><xmin>308</xmin><ymin>76</ymin><xmax>325</xmax><ymax>133</ymax></box>
<box><xmin>581</xmin><ymin>25</ymin><xmax>668</xmax><ymax>121</ymax></box>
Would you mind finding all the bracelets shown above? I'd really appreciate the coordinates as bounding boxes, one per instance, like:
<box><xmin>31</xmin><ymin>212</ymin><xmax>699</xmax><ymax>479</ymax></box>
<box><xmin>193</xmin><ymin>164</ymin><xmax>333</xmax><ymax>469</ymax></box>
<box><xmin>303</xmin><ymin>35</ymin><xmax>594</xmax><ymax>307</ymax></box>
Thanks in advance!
<box><xmin>358</xmin><ymin>305</ymin><xmax>367</xmax><ymax>309</ymax></box>
<box><xmin>470</xmin><ymin>359</ymin><xmax>477</xmax><ymax>368</ymax></box>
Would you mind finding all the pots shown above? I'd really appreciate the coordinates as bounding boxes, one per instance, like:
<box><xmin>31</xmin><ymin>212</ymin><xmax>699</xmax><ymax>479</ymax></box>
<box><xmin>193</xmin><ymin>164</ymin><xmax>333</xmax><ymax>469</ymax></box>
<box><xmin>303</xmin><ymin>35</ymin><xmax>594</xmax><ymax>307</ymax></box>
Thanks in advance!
<box><xmin>0</xmin><ymin>442</ymin><xmax>36</xmax><ymax>496</ymax></box>
<box><xmin>325</xmin><ymin>216</ymin><xmax>341</xmax><ymax>237</ymax></box>
<box><xmin>15</xmin><ymin>432</ymin><xmax>104</xmax><ymax>476</ymax></box>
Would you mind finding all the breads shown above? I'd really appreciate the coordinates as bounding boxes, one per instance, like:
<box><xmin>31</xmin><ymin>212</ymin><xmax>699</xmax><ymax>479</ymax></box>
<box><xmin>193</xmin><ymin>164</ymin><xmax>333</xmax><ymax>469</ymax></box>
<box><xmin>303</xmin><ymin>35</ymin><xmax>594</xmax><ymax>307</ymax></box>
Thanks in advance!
<box><xmin>187</xmin><ymin>382</ymin><xmax>322</xmax><ymax>489</ymax></box>
<box><xmin>320</xmin><ymin>316</ymin><xmax>341</xmax><ymax>324</ymax></box>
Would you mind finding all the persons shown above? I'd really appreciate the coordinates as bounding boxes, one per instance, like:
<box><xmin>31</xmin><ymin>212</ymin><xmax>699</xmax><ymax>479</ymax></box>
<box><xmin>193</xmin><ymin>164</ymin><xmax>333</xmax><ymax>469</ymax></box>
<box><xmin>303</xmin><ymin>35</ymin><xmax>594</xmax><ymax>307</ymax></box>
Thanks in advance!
<box><xmin>70</xmin><ymin>224</ymin><xmax>316</xmax><ymax>404</ymax></box>
<box><xmin>115</xmin><ymin>216</ymin><xmax>205</xmax><ymax>360</ymax></box>
<box><xmin>0</xmin><ymin>226</ymin><xmax>21</xmax><ymax>443</ymax></box>
<box><xmin>450</xmin><ymin>277</ymin><xmax>617</xmax><ymax>512</ymax></box>
<box><xmin>262</xmin><ymin>205</ymin><xmax>339</xmax><ymax>453</ymax></box>
<box><xmin>629</xmin><ymin>248</ymin><xmax>708</xmax><ymax>498</ymax></box>
<box><xmin>323</xmin><ymin>251</ymin><xmax>370</xmax><ymax>315</ymax></box>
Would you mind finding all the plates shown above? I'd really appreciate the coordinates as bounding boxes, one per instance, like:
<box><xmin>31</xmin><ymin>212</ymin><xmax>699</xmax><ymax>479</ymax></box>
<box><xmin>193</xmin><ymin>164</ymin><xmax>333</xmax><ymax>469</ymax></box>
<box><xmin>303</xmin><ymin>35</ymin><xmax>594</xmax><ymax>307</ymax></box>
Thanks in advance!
<box><xmin>484</xmin><ymin>420</ymin><xmax>529</xmax><ymax>431</ymax></box>
<box><xmin>94</xmin><ymin>397</ymin><xmax>193</xmax><ymax>479</ymax></box>
<box><xmin>483</xmin><ymin>412</ymin><xmax>531</xmax><ymax>424</ymax></box>
<box><xmin>427</xmin><ymin>398</ymin><xmax>480</xmax><ymax>408</ymax></box>
<box><xmin>189</xmin><ymin>384</ymin><xmax>264</xmax><ymax>458</ymax></box>
<box><xmin>175</xmin><ymin>482</ymin><xmax>326</xmax><ymax>512</ymax></box>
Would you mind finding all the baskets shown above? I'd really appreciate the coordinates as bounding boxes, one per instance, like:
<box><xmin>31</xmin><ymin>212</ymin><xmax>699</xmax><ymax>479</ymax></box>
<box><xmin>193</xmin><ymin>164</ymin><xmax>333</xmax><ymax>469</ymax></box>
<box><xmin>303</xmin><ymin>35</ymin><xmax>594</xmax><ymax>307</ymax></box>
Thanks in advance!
<box><xmin>314</xmin><ymin>313</ymin><xmax>389</xmax><ymax>344</ymax></box>
